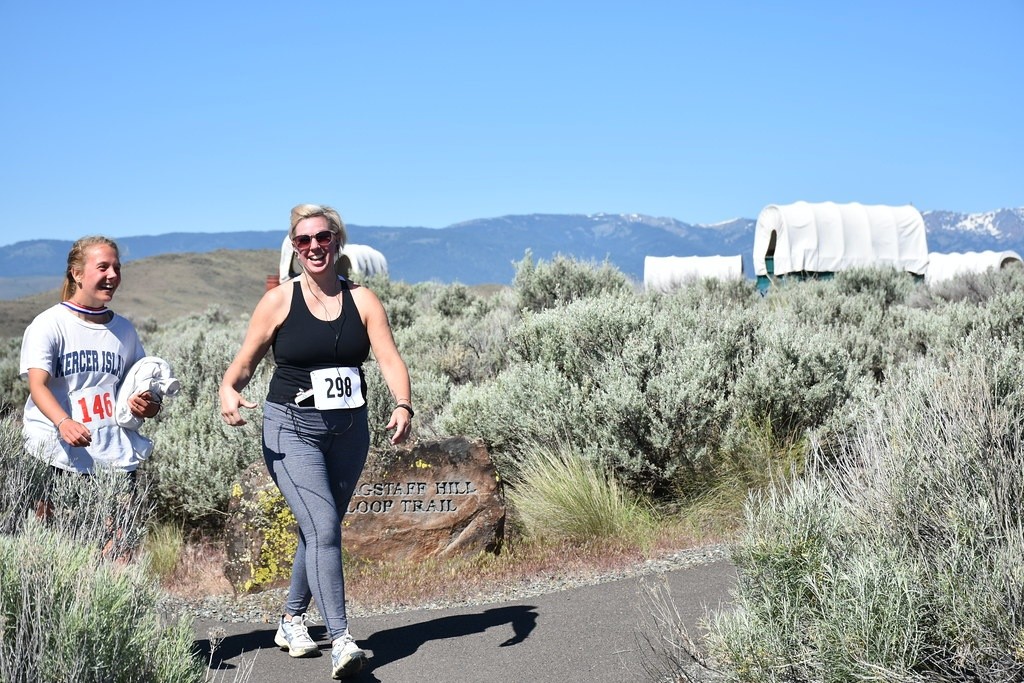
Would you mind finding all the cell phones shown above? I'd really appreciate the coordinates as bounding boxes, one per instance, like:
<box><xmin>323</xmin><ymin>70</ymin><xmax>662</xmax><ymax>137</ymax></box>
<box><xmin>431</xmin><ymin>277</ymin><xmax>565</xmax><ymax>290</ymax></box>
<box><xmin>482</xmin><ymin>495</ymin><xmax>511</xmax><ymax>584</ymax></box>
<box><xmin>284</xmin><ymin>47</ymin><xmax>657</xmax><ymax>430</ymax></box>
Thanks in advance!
<box><xmin>294</xmin><ymin>388</ymin><xmax>316</xmax><ymax>408</ymax></box>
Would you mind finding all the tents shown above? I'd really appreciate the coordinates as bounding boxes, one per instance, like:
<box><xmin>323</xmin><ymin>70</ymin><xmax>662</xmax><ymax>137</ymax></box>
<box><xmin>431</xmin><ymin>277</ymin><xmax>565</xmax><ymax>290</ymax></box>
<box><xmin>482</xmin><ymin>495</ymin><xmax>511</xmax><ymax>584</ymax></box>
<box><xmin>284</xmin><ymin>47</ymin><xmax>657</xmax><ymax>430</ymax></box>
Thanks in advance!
<box><xmin>276</xmin><ymin>235</ymin><xmax>388</xmax><ymax>285</ymax></box>
<box><xmin>643</xmin><ymin>201</ymin><xmax>1024</xmax><ymax>296</ymax></box>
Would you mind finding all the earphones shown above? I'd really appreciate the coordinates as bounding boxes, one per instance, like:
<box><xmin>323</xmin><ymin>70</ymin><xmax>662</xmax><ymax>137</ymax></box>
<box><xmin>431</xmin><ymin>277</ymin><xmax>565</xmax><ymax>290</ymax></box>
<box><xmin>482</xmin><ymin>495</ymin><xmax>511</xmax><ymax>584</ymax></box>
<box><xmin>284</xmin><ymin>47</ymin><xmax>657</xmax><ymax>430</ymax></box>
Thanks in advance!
<box><xmin>295</xmin><ymin>250</ymin><xmax>297</xmax><ymax>256</ymax></box>
<box><xmin>335</xmin><ymin>243</ymin><xmax>340</xmax><ymax>253</ymax></box>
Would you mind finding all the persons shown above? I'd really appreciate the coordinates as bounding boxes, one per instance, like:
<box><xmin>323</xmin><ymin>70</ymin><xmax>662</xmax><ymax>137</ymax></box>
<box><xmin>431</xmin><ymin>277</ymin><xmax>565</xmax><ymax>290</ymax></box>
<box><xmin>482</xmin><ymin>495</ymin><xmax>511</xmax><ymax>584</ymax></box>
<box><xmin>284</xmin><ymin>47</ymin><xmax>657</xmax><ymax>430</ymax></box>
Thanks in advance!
<box><xmin>217</xmin><ymin>203</ymin><xmax>414</xmax><ymax>679</ymax></box>
<box><xmin>18</xmin><ymin>234</ymin><xmax>180</xmax><ymax>562</ymax></box>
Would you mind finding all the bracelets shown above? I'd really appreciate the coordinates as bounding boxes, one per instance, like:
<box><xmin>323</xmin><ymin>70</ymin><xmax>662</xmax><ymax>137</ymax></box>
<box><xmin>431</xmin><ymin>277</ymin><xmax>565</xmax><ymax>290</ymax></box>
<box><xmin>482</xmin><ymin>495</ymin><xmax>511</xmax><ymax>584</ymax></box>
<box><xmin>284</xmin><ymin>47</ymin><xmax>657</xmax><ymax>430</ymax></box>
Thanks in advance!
<box><xmin>393</xmin><ymin>404</ymin><xmax>414</xmax><ymax>419</ymax></box>
<box><xmin>56</xmin><ymin>417</ymin><xmax>72</xmax><ymax>430</ymax></box>
<box><xmin>395</xmin><ymin>398</ymin><xmax>411</xmax><ymax>404</ymax></box>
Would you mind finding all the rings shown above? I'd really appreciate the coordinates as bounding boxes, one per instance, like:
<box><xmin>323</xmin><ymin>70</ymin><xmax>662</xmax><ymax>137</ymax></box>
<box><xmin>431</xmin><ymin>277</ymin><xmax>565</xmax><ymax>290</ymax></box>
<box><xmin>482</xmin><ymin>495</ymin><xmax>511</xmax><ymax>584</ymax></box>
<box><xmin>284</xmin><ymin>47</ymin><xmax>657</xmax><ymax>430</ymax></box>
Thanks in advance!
<box><xmin>136</xmin><ymin>404</ymin><xmax>139</xmax><ymax>408</ymax></box>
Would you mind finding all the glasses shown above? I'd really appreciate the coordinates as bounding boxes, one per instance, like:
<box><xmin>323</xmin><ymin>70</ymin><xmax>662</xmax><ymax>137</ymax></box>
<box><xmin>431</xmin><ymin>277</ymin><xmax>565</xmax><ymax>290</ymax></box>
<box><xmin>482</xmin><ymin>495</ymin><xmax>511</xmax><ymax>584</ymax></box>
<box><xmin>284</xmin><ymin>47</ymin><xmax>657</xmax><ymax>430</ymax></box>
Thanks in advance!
<box><xmin>292</xmin><ymin>230</ymin><xmax>336</xmax><ymax>250</ymax></box>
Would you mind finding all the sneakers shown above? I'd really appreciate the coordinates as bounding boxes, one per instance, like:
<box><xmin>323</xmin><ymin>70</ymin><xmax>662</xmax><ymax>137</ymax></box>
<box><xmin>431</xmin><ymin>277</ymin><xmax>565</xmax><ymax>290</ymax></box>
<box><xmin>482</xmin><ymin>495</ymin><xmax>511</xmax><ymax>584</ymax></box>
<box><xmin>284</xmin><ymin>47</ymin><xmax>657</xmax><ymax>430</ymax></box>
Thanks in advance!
<box><xmin>332</xmin><ymin>624</ymin><xmax>370</xmax><ymax>679</ymax></box>
<box><xmin>274</xmin><ymin>613</ymin><xmax>319</xmax><ymax>658</ymax></box>
